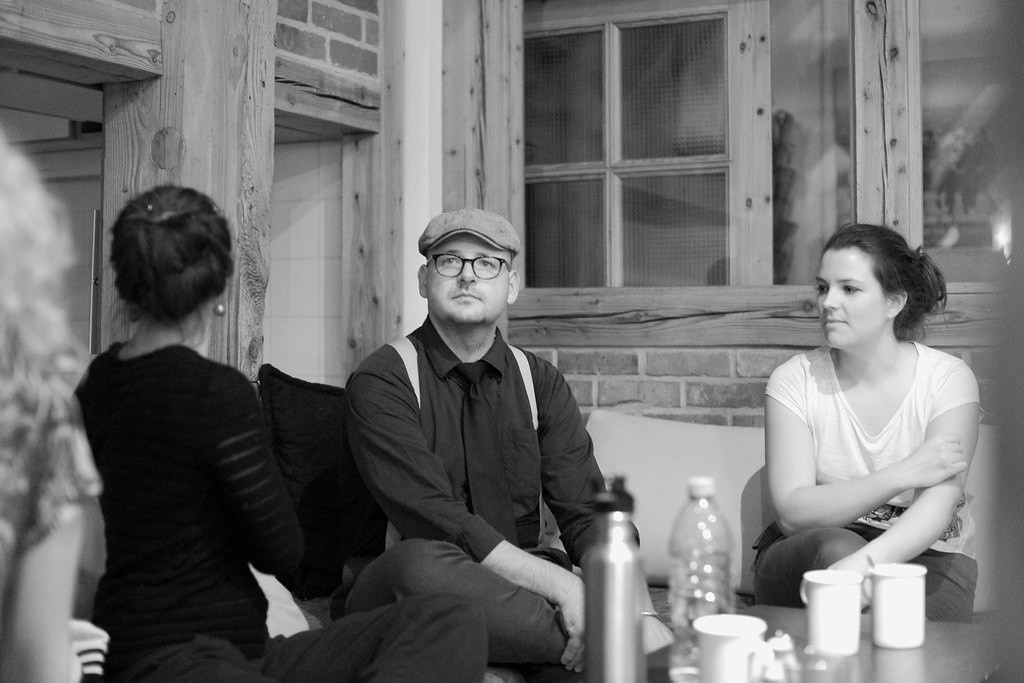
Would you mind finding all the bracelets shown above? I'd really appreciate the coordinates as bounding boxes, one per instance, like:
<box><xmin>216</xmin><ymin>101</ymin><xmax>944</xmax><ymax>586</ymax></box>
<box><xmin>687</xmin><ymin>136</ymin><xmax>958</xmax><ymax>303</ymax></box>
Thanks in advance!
<box><xmin>642</xmin><ymin>610</ymin><xmax>661</xmax><ymax>619</ymax></box>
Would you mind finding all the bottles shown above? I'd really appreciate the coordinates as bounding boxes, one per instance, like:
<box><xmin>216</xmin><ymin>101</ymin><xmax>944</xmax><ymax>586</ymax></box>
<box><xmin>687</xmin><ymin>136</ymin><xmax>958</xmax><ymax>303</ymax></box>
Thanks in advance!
<box><xmin>668</xmin><ymin>475</ymin><xmax>731</xmax><ymax>679</ymax></box>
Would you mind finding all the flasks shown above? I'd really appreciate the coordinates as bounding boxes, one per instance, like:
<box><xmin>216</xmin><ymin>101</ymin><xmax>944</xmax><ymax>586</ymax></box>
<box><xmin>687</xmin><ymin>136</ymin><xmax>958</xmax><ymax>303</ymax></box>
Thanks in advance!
<box><xmin>581</xmin><ymin>476</ymin><xmax>645</xmax><ymax>683</ymax></box>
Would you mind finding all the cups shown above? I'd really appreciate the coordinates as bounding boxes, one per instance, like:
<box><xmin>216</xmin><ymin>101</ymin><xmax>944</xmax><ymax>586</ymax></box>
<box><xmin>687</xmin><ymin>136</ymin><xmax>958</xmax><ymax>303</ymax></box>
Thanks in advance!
<box><xmin>862</xmin><ymin>563</ymin><xmax>927</xmax><ymax>649</ymax></box>
<box><xmin>800</xmin><ymin>569</ymin><xmax>864</xmax><ymax>656</ymax></box>
<box><xmin>693</xmin><ymin>614</ymin><xmax>775</xmax><ymax>683</ymax></box>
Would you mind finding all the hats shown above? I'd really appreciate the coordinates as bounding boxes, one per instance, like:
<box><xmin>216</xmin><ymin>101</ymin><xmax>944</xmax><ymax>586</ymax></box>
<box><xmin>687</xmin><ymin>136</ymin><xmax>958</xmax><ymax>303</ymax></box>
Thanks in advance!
<box><xmin>417</xmin><ymin>208</ymin><xmax>521</xmax><ymax>256</ymax></box>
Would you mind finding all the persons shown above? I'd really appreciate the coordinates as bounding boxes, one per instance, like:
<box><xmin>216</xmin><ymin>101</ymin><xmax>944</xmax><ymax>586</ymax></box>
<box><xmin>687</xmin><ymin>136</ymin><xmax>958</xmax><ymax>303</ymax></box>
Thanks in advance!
<box><xmin>346</xmin><ymin>208</ymin><xmax>674</xmax><ymax>683</ymax></box>
<box><xmin>72</xmin><ymin>187</ymin><xmax>490</xmax><ymax>683</ymax></box>
<box><xmin>0</xmin><ymin>146</ymin><xmax>109</xmax><ymax>683</ymax></box>
<box><xmin>754</xmin><ymin>224</ymin><xmax>982</xmax><ymax>624</ymax></box>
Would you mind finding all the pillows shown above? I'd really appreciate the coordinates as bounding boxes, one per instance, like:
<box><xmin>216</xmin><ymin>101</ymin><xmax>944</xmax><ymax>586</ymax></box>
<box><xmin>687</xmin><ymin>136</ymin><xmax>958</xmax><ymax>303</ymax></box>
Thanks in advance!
<box><xmin>255</xmin><ymin>362</ymin><xmax>389</xmax><ymax>602</ymax></box>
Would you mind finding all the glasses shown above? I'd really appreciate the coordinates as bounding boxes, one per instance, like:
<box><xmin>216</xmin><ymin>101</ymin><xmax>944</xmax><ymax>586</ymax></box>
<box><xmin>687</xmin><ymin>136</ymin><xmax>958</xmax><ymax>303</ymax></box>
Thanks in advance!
<box><xmin>425</xmin><ymin>252</ymin><xmax>512</xmax><ymax>280</ymax></box>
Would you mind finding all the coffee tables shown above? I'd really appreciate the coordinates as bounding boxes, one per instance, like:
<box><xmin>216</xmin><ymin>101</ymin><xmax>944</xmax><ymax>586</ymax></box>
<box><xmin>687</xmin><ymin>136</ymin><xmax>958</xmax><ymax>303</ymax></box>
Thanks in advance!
<box><xmin>742</xmin><ymin>605</ymin><xmax>1023</xmax><ymax>683</ymax></box>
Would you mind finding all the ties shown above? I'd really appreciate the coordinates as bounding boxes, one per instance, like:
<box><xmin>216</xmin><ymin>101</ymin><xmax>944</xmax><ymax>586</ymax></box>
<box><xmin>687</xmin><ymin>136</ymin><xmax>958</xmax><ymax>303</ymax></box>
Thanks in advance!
<box><xmin>454</xmin><ymin>362</ymin><xmax>522</xmax><ymax>547</ymax></box>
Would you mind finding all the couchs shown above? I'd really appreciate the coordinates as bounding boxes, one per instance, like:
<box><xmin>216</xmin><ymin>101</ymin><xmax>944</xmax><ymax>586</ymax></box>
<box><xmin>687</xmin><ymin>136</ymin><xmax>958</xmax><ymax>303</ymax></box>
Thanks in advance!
<box><xmin>72</xmin><ymin>383</ymin><xmax>1023</xmax><ymax>683</ymax></box>
